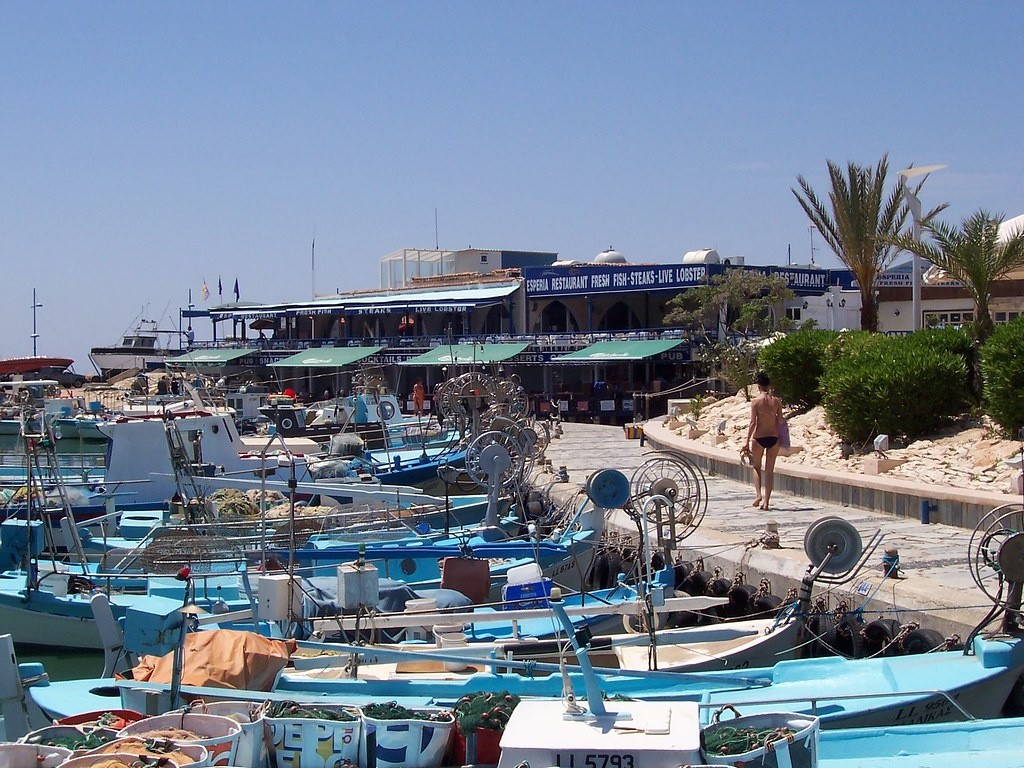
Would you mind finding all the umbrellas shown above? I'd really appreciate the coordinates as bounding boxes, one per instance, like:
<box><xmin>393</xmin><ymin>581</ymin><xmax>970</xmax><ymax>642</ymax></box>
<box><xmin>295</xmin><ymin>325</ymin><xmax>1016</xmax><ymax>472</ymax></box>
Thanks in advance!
<box><xmin>923</xmin><ymin>212</ymin><xmax>1024</xmax><ymax>284</ymax></box>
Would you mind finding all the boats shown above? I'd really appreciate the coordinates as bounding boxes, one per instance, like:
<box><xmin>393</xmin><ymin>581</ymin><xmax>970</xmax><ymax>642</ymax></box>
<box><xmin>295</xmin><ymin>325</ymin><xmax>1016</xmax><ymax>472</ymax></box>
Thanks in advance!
<box><xmin>0</xmin><ymin>275</ymin><xmax>1024</xmax><ymax>767</ymax></box>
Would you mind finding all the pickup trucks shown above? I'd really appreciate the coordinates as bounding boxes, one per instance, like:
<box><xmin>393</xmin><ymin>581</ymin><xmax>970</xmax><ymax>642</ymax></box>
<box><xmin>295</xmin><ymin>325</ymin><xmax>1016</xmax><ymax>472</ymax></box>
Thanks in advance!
<box><xmin>24</xmin><ymin>364</ymin><xmax>86</xmax><ymax>388</ymax></box>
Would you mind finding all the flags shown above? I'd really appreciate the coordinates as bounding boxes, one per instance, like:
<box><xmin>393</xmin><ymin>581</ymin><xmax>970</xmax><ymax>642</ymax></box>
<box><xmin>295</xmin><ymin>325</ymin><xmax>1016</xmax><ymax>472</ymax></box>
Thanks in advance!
<box><xmin>202</xmin><ymin>277</ymin><xmax>210</xmax><ymax>300</ymax></box>
<box><xmin>233</xmin><ymin>278</ymin><xmax>240</xmax><ymax>302</ymax></box>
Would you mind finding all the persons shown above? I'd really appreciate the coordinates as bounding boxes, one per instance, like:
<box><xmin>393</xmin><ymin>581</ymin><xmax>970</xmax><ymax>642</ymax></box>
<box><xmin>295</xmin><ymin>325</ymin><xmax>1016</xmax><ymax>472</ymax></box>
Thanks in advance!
<box><xmin>739</xmin><ymin>372</ymin><xmax>783</xmax><ymax>510</ymax></box>
<box><xmin>413</xmin><ymin>377</ymin><xmax>424</xmax><ymax>418</ymax></box>
<box><xmin>158</xmin><ymin>376</ymin><xmax>179</xmax><ymax>395</ymax></box>
<box><xmin>299</xmin><ymin>386</ymin><xmax>332</xmax><ymax>402</ymax></box>
<box><xmin>183</xmin><ymin>326</ymin><xmax>195</xmax><ymax>346</ymax></box>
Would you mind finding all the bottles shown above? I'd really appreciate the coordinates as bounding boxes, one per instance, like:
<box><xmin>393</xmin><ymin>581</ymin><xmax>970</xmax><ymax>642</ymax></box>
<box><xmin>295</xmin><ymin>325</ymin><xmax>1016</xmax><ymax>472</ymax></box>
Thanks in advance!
<box><xmin>358</xmin><ymin>540</ymin><xmax>367</xmax><ymax>568</ymax></box>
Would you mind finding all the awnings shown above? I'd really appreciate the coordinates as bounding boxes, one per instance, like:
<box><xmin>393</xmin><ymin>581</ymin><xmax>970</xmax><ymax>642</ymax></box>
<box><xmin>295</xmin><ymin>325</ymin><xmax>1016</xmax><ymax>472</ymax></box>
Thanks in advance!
<box><xmin>163</xmin><ymin>349</ymin><xmax>259</xmax><ymax>362</ymax></box>
<box><xmin>267</xmin><ymin>348</ymin><xmax>385</xmax><ymax>366</ymax></box>
<box><xmin>552</xmin><ymin>339</ymin><xmax>685</xmax><ymax>360</ymax></box>
<box><xmin>209</xmin><ymin>298</ymin><xmax>503</xmax><ymax>320</ymax></box>
<box><xmin>398</xmin><ymin>344</ymin><xmax>529</xmax><ymax>364</ymax></box>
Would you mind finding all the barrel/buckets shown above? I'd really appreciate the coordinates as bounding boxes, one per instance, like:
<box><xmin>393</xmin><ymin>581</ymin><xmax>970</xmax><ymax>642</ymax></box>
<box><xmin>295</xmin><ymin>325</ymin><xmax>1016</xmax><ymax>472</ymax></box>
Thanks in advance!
<box><xmin>89</xmin><ymin>401</ymin><xmax>101</xmax><ymax>413</ymax></box>
<box><xmin>440</xmin><ymin>633</ymin><xmax>469</xmax><ymax>672</ymax></box>
<box><xmin>238</xmin><ymin>568</ymin><xmax>269</xmax><ymax>600</ymax></box>
<box><xmin>404</xmin><ymin>598</ymin><xmax>441</xmax><ymax>643</ymax></box>
<box><xmin>170</xmin><ymin>513</ymin><xmax>188</xmax><ymax>526</ymax></box>
<box><xmin>432</xmin><ymin>624</ymin><xmax>464</xmax><ymax>647</ymax></box>
<box><xmin>38</xmin><ymin>564</ymin><xmax>69</xmax><ymax>596</ymax></box>
